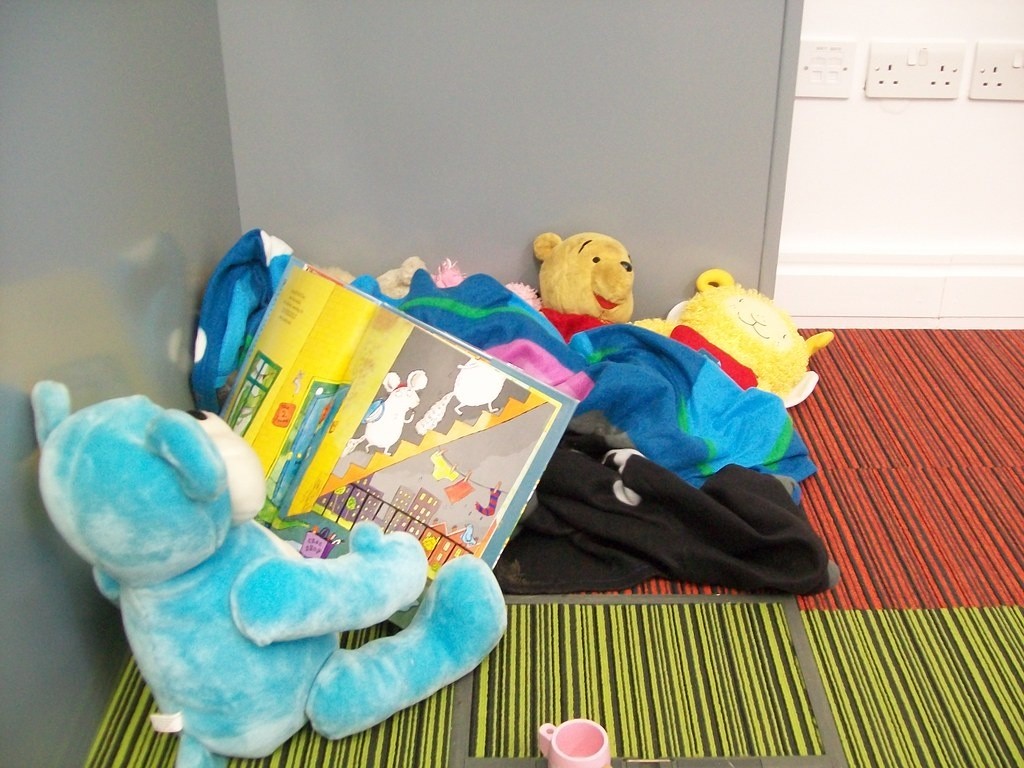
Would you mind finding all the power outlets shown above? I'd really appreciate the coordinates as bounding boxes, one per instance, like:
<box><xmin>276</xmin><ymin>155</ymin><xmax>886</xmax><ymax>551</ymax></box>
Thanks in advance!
<box><xmin>969</xmin><ymin>40</ymin><xmax>1024</xmax><ymax>102</ymax></box>
<box><xmin>866</xmin><ymin>39</ymin><xmax>964</xmax><ymax>100</ymax></box>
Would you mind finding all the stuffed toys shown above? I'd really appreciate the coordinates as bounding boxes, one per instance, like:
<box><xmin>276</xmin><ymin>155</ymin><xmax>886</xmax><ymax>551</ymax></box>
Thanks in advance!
<box><xmin>531</xmin><ymin>232</ymin><xmax>633</xmax><ymax>345</ymax></box>
<box><xmin>625</xmin><ymin>270</ymin><xmax>834</xmax><ymax>409</ymax></box>
<box><xmin>32</xmin><ymin>379</ymin><xmax>508</xmax><ymax>767</ymax></box>
<box><xmin>325</xmin><ymin>256</ymin><xmax>548</xmax><ymax>395</ymax></box>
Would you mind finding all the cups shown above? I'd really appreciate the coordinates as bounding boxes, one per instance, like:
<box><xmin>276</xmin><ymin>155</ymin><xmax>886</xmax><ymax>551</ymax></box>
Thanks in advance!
<box><xmin>538</xmin><ymin>719</ymin><xmax>611</xmax><ymax>768</ymax></box>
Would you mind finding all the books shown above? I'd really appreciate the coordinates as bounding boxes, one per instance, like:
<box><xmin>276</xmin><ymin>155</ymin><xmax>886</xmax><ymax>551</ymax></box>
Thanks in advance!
<box><xmin>224</xmin><ymin>258</ymin><xmax>581</xmax><ymax>632</ymax></box>
